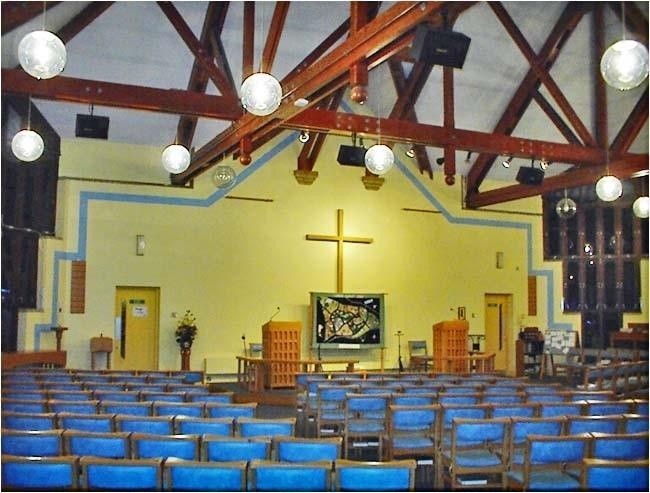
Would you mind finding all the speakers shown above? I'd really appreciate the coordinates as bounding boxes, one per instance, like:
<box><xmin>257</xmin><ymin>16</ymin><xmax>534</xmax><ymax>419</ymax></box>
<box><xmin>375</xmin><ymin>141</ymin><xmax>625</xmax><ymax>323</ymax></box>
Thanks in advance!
<box><xmin>336</xmin><ymin>145</ymin><xmax>367</xmax><ymax>167</ymax></box>
<box><xmin>75</xmin><ymin>114</ymin><xmax>110</xmax><ymax>139</ymax></box>
<box><xmin>516</xmin><ymin>166</ymin><xmax>545</xmax><ymax>186</ymax></box>
<box><xmin>409</xmin><ymin>25</ymin><xmax>471</xmax><ymax>69</ymax></box>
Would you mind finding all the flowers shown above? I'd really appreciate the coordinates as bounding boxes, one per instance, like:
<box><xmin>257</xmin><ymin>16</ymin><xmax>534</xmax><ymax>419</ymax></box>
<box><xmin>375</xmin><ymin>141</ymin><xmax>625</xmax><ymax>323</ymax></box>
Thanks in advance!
<box><xmin>175</xmin><ymin>309</ymin><xmax>198</xmax><ymax>342</ymax></box>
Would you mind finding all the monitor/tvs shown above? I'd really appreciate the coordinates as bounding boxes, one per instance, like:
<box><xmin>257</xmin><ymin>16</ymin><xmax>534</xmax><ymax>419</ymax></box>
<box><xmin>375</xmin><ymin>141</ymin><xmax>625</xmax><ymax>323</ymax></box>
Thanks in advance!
<box><xmin>309</xmin><ymin>291</ymin><xmax>387</xmax><ymax>351</ymax></box>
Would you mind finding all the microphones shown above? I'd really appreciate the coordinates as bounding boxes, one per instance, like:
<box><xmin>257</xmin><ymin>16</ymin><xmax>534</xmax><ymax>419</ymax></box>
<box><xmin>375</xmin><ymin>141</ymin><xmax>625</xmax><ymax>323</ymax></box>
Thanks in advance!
<box><xmin>449</xmin><ymin>307</ymin><xmax>466</xmax><ymax>321</ymax></box>
<box><xmin>268</xmin><ymin>305</ymin><xmax>281</xmax><ymax>320</ymax></box>
